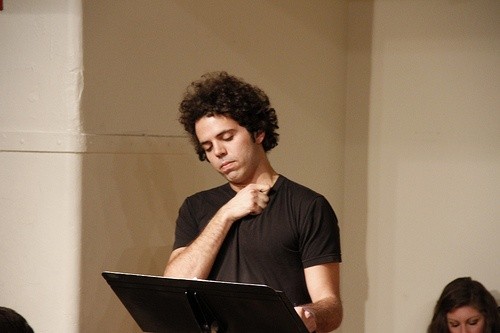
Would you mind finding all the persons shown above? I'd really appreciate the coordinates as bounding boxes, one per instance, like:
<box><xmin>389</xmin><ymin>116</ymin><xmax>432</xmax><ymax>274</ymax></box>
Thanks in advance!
<box><xmin>423</xmin><ymin>276</ymin><xmax>500</xmax><ymax>333</ymax></box>
<box><xmin>162</xmin><ymin>70</ymin><xmax>345</xmax><ymax>333</ymax></box>
<box><xmin>0</xmin><ymin>306</ymin><xmax>35</xmax><ymax>333</ymax></box>
